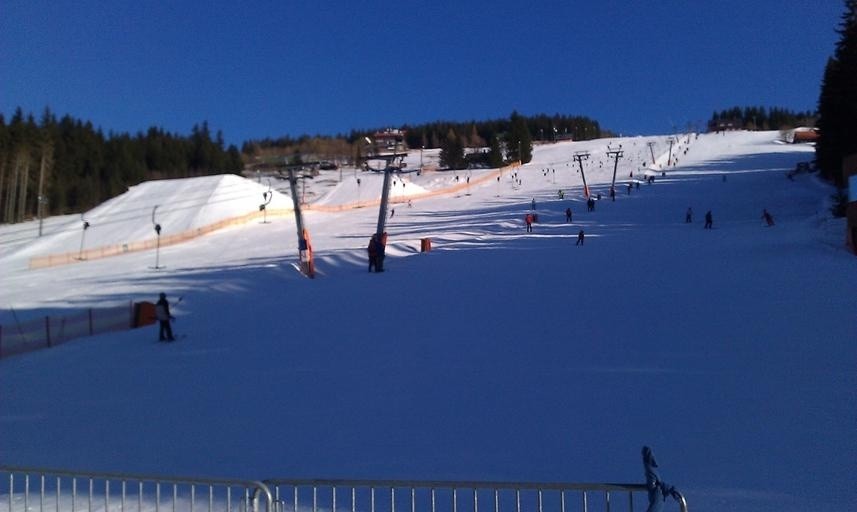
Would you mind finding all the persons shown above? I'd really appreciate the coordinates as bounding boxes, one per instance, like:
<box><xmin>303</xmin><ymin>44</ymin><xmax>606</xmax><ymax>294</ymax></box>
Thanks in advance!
<box><xmin>524</xmin><ymin>213</ymin><xmax>532</xmax><ymax>232</ymax></box>
<box><xmin>685</xmin><ymin>208</ymin><xmax>692</xmax><ymax>223</ymax></box>
<box><xmin>531</xmin><ymin>198</ymin><xmax>536</xmax><ymax>210</ymax></box>
<box><xmin>153</xmin><ymin>292</ymin><xmax>177</xmax><ymax>342</ymax></box>
<box><xmin>760</xmin><ymin>209</ymin><xmax>774</xmax><ymax>227</ymax></box>
<box><xmin>565</xmin><ymin>208</ymin><xmax>571</xmax><ymax>222</ymax></box>
<box><xmin>576</xmin><ymin>230</ymin><xmax>584</xmax><ymax>246</ymax></box>
<box><xmin>585</xmin><ymin>175</ymin><xmax>658</xmax><ymax>212</ymax></box>
<box><xmin>366</xmin><ymin>236</ymin><xmax>383</xmax><ymax>273</ymax></box>
<box><xmin>703</xmin><ymin>210</ymin><xmax>712</xmax><ymax>229</ymax></box>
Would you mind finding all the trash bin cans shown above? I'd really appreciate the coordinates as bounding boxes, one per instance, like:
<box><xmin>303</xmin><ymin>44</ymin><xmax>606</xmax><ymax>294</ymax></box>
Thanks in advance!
<box><xmin>598</xmin><ymin>194</ymin><xmax>601</xmax><ymax>200</ymax></box>
<box><xmin>133</xmin><ymin>300</ymin><xmax>158</xmax><ymax>328</ymax></box>
<box><xmin>421</xmin><ymin>238</ymin><xmax>431</xmax><ymax>252</ymax></box>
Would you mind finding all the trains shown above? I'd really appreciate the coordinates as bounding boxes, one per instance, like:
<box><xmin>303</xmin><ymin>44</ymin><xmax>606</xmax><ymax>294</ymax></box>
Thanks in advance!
<box><xmin>254</xmin><ymin>161</ymin><xmax>337</xmax><ymax>172</ymax></box>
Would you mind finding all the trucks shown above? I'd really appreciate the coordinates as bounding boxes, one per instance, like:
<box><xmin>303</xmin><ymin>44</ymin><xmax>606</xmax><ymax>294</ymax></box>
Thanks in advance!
<box><xmin>352</xmin><ymin>133</ymin><xmax>408</xmax><ymax>157</ymax></box>
